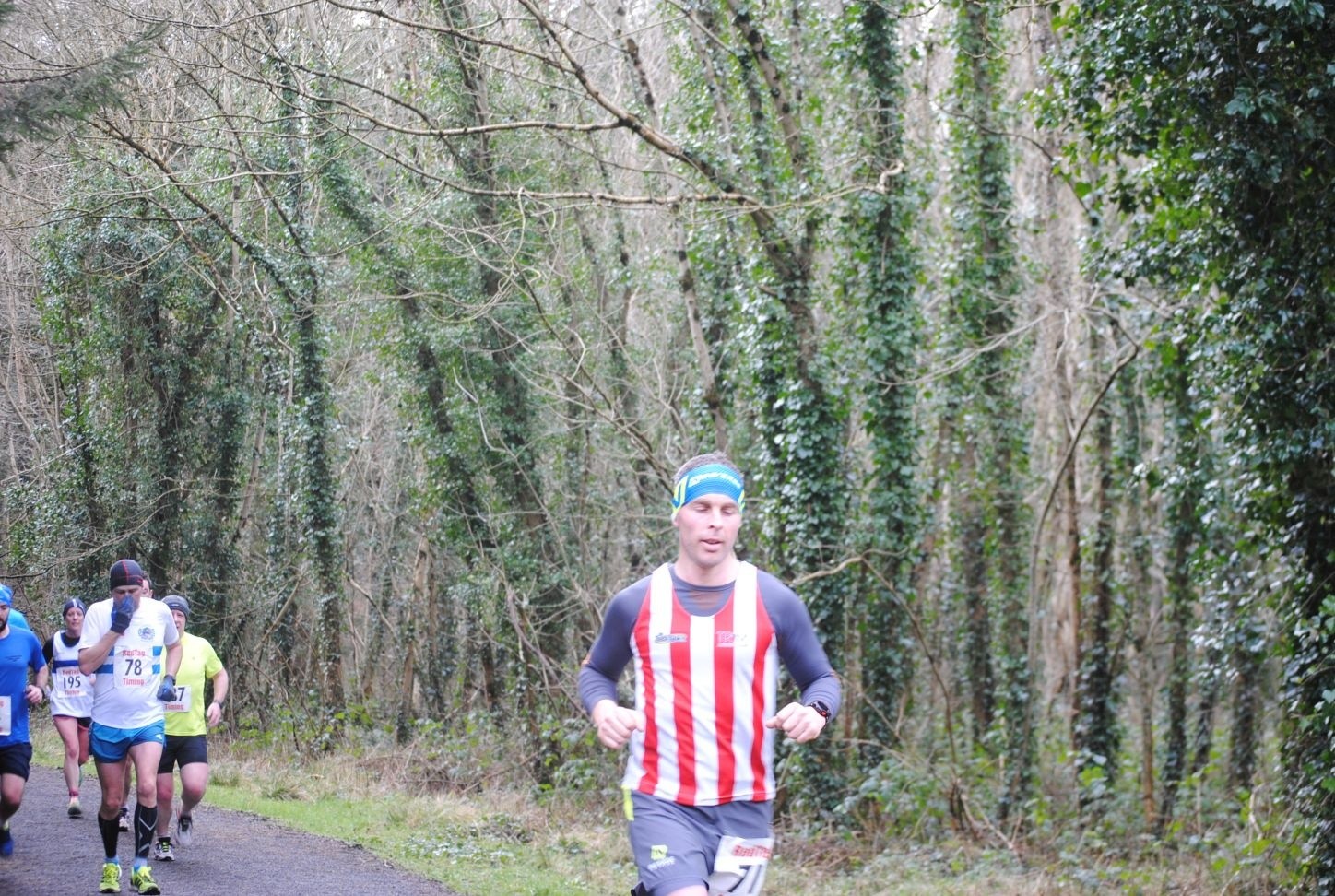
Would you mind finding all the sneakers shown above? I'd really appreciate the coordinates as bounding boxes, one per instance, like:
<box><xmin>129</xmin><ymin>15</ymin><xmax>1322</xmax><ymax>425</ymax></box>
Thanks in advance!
<box><xmin>68</xmin><ymin>793</ymin><xmax>82</xmax><ymax>817</ymax></box>
<box><xmin>99</xmin><ymin>862</ymin><xmax>122</xmax><ymax>892</ymax></box>
<box><xmin>119</xmin><ymin>807</ymin><xmax>130</xmax><ymax>831</ymax></box>
<box><xmin>154</xmin><ymin>837</ymin><xmax>174</xmax><ymax>861</ymax></box>
<box><xmin>129</xmin><ymin>865</ymin><xmax>161</xmax><ymax>895</ymax></box>
<box><xmin>0</xmin><ymin>823</ymin><xmax>13</xmax><ymax>857</ymax></box>
<box><xmin>79</xmin><ymin>764</ymin><xmax>82</xmax><ymax>786</ymax></box>
<box><xmin>176</xmin><ymin>802</ymin><xmax>194</xmax><ymax>847</ymax></box>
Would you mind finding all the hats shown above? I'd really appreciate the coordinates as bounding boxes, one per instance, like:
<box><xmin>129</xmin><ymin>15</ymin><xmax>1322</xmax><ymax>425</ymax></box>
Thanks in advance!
<box><xmin>63</xmin><ymin>599</ymin><xmax>86</xmax><ymax>619</ymax></box>
<box><xmin>0</xmin><ymin>584</ymin><xmax>13</xmax><ymax>607</ymax></box>
<box><xmin>161</xmin><ymin>595</ymin><xmax>188</xmax><ymax>620</ymax></box>
<box><xmin>110</xmin><ymin>560</ymin><xmax>143</xmax><ymax>590</ymax></box>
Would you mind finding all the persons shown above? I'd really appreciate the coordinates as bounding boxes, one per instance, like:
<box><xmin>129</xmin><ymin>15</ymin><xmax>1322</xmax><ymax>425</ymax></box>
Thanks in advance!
<box><xmin>577</xmin><ymin>451</ymin><xmax>843</xmax><ymax>896</ymax></box>
<box><xmin>0</xmin><ymin>584</ymin><xmax>49</xmax><ymax>860</ymax></box>
<box><xmin>118</xmin><ymin>571</ymin><xmax>153</xmax><ymax>831</ymax></box>
<box><xmin>154</xmin><ymin>594</ymin><xmax>230</xmax><ymax>861</ymax></box>
<box><xmin>77</xmin><ymin>560</ymin><xmax>183</xmax><ymax>896</ymax></box>
<box><xmin>41</xmin><ymin>599</ymin><xmax>96</xmax><ymax>817</ymax></box>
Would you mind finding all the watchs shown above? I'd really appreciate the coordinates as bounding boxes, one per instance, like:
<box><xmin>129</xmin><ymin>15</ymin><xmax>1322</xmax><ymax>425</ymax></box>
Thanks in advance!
<box><xmin>37</xmin><ymin>685</ymin><xmax>46</xmax><ymax>701</ymax></box>
<box><xmin>213</xmin><ymin>700</ymin><xmax>224</xmax><ymax>710</ymax></box>
<box><xmin>807</xmin><ymin>701</ymin><xmax>830</xmax><ymax>724</ymax></box>
<box><xmin>164</xmin><ymin>674</ymin><xmax>176</xmax><ymax>686</ymax></box>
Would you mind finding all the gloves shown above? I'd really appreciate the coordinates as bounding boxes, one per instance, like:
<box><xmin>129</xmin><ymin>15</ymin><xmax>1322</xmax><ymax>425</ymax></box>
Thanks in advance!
<box><xmin>110</xmin><ymin>594</ymin><xmax>134</xmax><ymax>633</ymax></box>
<box><xmin>157</xmin><ymin>675</ymin><xmax>177</xmax><ymax>701</ymax></box>
